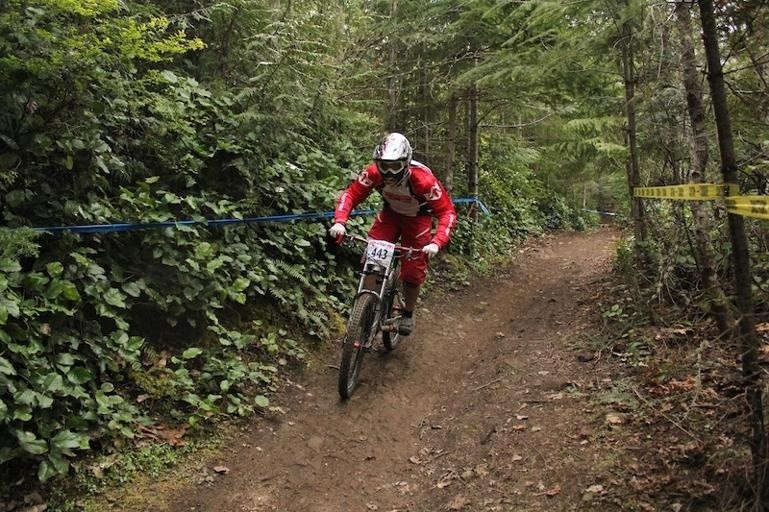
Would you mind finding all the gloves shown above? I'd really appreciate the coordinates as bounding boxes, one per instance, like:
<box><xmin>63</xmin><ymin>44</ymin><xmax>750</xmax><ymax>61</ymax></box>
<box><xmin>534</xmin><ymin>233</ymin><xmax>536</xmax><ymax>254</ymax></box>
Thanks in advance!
<box><xmin>328</xmin><ymin>222</ymin><xmax>349</xmax><ymax>238</ymax></box>
<box><xmin>421</xmin><ymin>241</ymin><xmax>440</xmax><ymax>259</ymax></box>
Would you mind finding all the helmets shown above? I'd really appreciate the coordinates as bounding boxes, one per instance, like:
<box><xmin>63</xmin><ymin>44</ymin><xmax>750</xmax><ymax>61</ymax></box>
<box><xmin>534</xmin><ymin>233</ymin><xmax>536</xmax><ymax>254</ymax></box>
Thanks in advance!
<box><xmin>373</xmin><ymin>132</ymin><xmax>412</xmax><ymax>161</ymax></box>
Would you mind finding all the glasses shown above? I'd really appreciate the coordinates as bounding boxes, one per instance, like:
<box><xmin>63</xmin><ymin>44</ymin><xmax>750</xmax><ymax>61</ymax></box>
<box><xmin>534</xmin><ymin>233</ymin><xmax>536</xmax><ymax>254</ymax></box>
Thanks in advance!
<box><xmin>376</xmin><ymin>162</ymin><xmax>406</xmax><ymax>175</ymax></box>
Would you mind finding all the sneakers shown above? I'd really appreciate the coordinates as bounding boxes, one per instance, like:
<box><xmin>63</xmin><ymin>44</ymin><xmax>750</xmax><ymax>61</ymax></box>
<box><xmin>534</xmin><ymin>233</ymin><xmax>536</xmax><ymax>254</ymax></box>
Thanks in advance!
<box><xmin>400</xmin><ymin>309</ymin><xmax>415</xmax><ymax>336</ymax></box>
<box><xmin>358</xmin><ymin>321</ymin><xmax>379</xmax><ymax>348</ymax></box>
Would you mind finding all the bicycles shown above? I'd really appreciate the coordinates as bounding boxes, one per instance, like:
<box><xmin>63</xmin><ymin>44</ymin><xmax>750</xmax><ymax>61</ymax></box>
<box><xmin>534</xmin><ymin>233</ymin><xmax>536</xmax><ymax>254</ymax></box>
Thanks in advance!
<box><xmin>328</xmin><ymin>229</ymin><xmax>423</xmax><ymax>399</ymax></box>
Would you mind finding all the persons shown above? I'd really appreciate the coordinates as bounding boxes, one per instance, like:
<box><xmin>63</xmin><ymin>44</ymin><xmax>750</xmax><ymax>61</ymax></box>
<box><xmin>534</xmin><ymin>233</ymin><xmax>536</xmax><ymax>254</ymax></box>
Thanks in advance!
<box><xmin>328</xmin><ymin>130</ymin><xmax>459</xmax><ymax>339</ymax></box>
